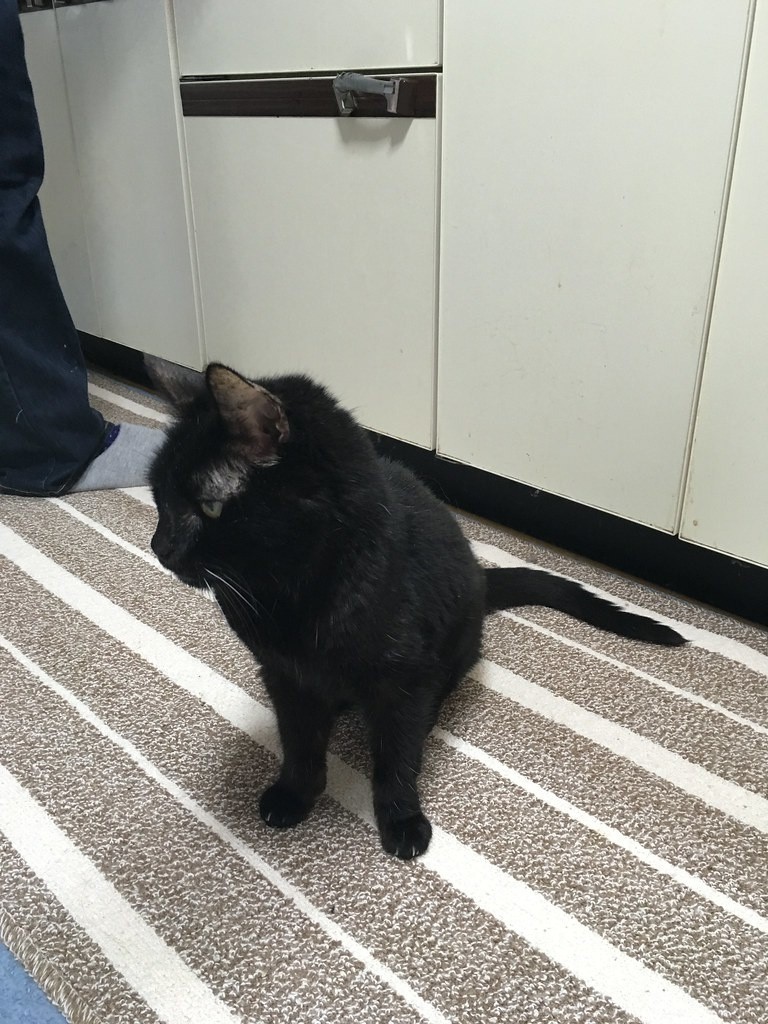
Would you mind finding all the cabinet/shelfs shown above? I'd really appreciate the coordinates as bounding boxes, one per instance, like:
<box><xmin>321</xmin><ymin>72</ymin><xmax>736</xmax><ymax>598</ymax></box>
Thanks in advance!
<box><xmin>8</xmin><ymin>0</ymin><xmax>767</xmax><ymax>623</ymax></box>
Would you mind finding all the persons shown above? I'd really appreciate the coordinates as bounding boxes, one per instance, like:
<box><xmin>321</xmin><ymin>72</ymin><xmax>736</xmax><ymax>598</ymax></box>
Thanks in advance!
<box><xmin>0</xmin><ymin>4</ymin><xmax>173</xmax><ymax>497</ymax></box>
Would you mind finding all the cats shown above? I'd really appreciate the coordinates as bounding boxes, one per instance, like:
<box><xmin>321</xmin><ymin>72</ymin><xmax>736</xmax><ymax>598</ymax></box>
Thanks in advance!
<box><xmin>142</xmin><ymin>348</ymin><xmax>693</xmax><ymax>859</ymax></box>
